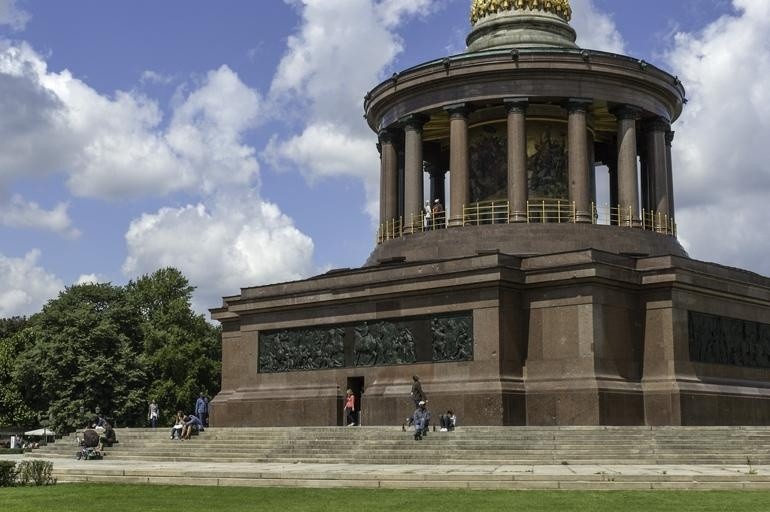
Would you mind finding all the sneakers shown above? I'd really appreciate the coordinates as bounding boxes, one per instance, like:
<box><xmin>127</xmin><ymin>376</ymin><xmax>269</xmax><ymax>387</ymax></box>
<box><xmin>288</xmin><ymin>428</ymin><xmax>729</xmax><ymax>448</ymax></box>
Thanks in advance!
<box><xmin>169</xmin><ymin>435</ymin><xmax>191</xmax><ymax>439</ymax></box>
<box><xmin>440</xmin><ymin>428</ymin><xmax>448</xmax><ymax>432</ymax></box>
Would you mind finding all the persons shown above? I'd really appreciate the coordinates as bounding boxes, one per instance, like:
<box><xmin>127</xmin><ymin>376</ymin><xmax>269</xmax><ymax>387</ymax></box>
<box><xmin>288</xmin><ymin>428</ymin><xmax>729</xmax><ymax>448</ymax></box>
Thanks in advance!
<box><xmin>410</xmin><ymin>375</ymin><xmax>457</xmax><ymax>440</ymax></box>
<box><xmin>170</xmin><ymin>391</ymin><xmax>210</xmax><ymax>440</ymax></box>
<box><xmin>147</xmin><ymin>398</ymin><xmax>159</xmax><ymax>428</ymax></box>
<box><xmin>343</xmin><ymin>388</ymin><xmax>357</xmax><ymax>426</ymax></box>
<box><xmin>99</xmin><ymin>422</ymin><xmax>117</xmax><ymax>451</ymax></box>
<box><xmin>425</xmin><ymin>199</ymin><xmax>445</xmax><ymax>231</ymax></box>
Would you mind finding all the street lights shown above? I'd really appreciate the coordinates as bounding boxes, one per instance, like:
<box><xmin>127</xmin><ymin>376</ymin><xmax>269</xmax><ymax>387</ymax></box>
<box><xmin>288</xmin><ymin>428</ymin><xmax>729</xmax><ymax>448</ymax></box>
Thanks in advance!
<box><xmin>80</xmin><ymin>405</ymin><xmax>100</xmax><ymax>429</ymax></box>
<box><xmin>38</xmin><ymin>413</ymin><xmax>53</xmax><ymax>443</ymax></box>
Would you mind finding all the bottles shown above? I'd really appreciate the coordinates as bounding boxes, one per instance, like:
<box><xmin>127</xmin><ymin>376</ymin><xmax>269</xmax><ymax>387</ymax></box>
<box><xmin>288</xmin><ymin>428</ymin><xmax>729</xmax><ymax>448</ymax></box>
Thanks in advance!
<box><xmin>433</xmin><ymin>424</ymin><xmax>436</xmax><ymax>431</ymax></box>
<box><xmin>402</xmin><ymin>424</ymin><xmax>405</xmax><ymax>432</ymax></box>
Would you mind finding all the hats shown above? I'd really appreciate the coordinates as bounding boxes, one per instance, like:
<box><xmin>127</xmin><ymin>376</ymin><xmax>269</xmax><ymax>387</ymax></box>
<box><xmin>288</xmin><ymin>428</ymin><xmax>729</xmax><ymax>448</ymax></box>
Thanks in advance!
<box><xmin>419</xmin><ymin>401</ymin><xmax>425</xmax><ymax>405</ymax></box>
